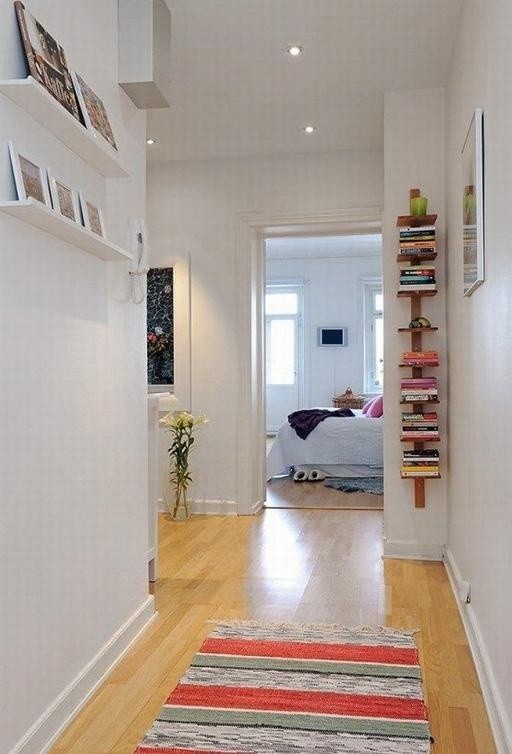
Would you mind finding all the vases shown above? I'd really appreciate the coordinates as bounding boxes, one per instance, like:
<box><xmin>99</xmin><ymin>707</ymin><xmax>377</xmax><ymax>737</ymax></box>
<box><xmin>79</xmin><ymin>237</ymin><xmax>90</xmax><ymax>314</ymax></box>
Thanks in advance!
<box><xmin>163</xmin><ymin>488</ymin><xmax>195</xmax><ymax>521</ymax></box>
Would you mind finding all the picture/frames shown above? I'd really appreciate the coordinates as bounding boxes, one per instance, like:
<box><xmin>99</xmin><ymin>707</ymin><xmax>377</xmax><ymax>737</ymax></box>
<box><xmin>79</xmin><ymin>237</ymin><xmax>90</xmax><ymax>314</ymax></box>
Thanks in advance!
<box><xmin>148</xmin><ymin>250</ymin><xmax>192</xmax><ymax>412</ymax></box>
<box><xmin>461</xmin><ymin>107</ymin><xmax>484</xmax><ymax>297</ymax></box>
<box><xmin>8</xmin><ymin>140</ymin><xmax>106</xmax><ymax>238</ymax></box>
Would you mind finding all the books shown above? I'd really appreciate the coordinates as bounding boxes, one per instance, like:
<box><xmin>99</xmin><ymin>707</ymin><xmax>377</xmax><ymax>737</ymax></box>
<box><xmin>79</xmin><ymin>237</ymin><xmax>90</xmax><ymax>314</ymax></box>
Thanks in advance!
<box><xmin>399</xmin><ymin>225</ymin><xmax>436</xmax><ymax>291</ymax></box>
<box><xmin>14</xmin><ymin>1</ymin><xmax>81</xmax><ymax>122</ymax></box>
<box><xmin>400</xmin><ymin>351</ymin><xmax>440</xmax><ymax>477</ymax></box>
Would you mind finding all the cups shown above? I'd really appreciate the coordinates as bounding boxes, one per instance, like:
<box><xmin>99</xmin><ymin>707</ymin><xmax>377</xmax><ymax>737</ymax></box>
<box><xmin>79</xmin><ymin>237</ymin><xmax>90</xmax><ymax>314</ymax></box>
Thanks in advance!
<box><xmin>410</xmin><ymin>197</ymin><xmax>428</xmax><ymax>218</ymax></box>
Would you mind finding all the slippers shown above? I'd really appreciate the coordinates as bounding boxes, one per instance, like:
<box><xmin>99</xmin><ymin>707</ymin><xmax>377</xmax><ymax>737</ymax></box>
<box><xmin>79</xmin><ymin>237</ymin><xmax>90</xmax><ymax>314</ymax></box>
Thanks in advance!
<box><xmin>307</xmin><ymin>470</ymin><xmax>325</xmax><ymax>481</ymax></box>
<box><xmin>293</xmin><ymin>470</ymin><xmax>308</xmax><ymax>482</ymax></box>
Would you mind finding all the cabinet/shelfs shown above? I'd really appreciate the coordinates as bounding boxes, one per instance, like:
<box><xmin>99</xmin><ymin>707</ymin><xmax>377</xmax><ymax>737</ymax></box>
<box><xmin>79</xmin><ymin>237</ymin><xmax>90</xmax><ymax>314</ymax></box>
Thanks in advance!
<box><xmin>396</xmin><ymin>188</ymin><xmax>441</xmax><ymax>509</ymax></box>
<box><xmin>0</xmin><ymin>79</ymin><xmax>134</xmax><ymax>261</ymax></box>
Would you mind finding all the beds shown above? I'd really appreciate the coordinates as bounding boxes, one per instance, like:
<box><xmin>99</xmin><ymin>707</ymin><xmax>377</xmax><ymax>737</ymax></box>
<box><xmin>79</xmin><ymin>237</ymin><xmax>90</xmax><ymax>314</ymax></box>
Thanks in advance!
<box><xmin>266</xmin><ymin>407</ymin><xmax>383</xmax><ymax>480</ymax></box>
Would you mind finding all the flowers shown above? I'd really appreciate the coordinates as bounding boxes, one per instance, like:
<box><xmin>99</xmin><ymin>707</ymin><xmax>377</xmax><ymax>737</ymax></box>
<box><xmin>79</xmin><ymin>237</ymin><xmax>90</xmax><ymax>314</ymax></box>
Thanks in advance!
<box><xmin>159</xmin><ymin>412</ymin><xmax>210</xmax><ymax>516</ymax></box>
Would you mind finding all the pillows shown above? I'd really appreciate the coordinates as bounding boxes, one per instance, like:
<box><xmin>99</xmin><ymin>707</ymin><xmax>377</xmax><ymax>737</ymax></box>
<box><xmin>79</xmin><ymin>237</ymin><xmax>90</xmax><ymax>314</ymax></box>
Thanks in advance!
<box><xmin>362</xmin><ymin>398</ymin><xmax>375</xmax><ymax>414</ymax></box>
<box><xmin>367</xmin><ymin>396</ymin><xmax>383</xmax><ymax>417</ymax></box>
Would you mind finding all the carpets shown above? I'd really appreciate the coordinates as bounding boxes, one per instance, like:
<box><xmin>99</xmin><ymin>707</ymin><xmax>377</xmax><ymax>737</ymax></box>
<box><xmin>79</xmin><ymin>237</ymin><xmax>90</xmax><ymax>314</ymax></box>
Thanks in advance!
<box><xmin>133</xmin><ymin>617</ymin><xmax>432</xmax><ymax>753</ymax></box>
